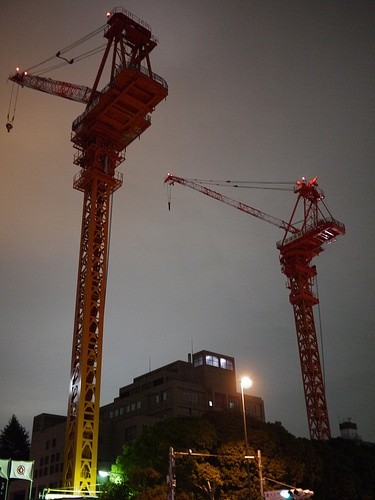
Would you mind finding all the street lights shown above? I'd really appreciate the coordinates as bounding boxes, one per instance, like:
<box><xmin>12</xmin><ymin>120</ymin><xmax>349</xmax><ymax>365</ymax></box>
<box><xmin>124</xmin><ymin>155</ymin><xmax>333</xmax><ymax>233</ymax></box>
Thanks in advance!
<box><xmin>240</xmin><ymin>376</ymin><xmax>264</xmax><ymax>499</ymax></box>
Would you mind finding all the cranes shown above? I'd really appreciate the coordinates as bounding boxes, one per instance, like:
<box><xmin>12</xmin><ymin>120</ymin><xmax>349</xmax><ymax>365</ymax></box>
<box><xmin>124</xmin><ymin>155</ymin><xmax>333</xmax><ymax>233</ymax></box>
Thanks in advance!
<box><xmin>160</xmin><ymin>158</ymin><xmax>350</xmax><ymax>446</ymax></box>
<box><xmin>6</xmin><ymin>5</ymin><xmax>168</xmax><ymax>500</ymax></box>
